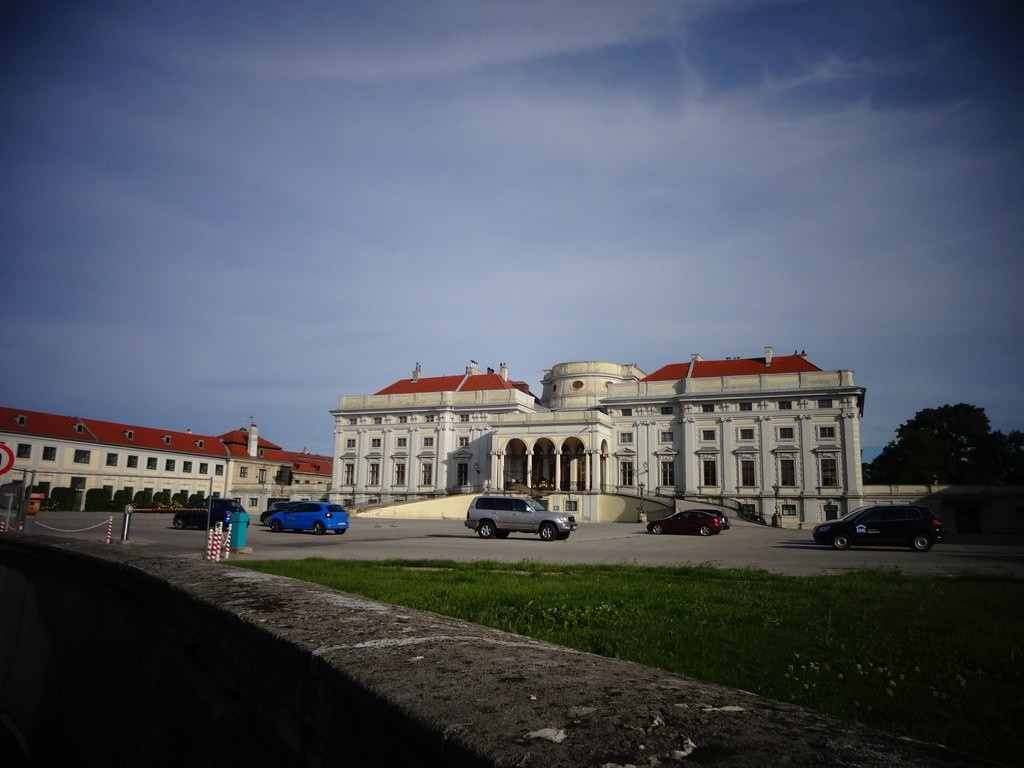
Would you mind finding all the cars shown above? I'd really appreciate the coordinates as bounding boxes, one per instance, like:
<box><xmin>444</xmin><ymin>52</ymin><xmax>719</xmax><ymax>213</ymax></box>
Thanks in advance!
<box><xmin>270</xmin><ymin>502</ymin><xmax>349</xmax><ymax>536</ymax></box>
<box><xmin>685</xmin><ymin>509</ymin><xmax>730</xmax><ymax>535</ymax></box>
<box><xmin>259</xmin><ymin>500</ymin><xmax>308</xmax><ymax>526</ymax></box>
<box><xmin>174</xmin><ymin>498</ymin><xmax>249</xmax><ymax>532</ymax></box>
<box><xmin>812</xmin><ymin>504</ymin><xmax>945</xmax><ymax>552</ymax></box>
<box><xmin>646</xmin><ymin>512</ymin><xmax>722</xmax><ymax>537</ymax></box>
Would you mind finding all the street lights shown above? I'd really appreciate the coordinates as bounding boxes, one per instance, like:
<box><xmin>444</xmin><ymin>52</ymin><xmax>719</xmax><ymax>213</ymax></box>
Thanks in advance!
<box><xmin>637</xmin><ymin>481</ymin><xmax>647</xmax><ymax>523</ymax></box>
<box><xmin>771</xmin><ymin>483</ymin><xmax>783</xmax><ymax>529</ymax></box>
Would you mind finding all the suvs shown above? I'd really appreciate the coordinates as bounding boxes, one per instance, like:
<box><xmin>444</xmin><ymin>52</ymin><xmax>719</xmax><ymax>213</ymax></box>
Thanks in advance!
<box><xmin>463</xmin><ymin>490</ymin><xmax>578</xmax><ymax>542</ymax></box>
